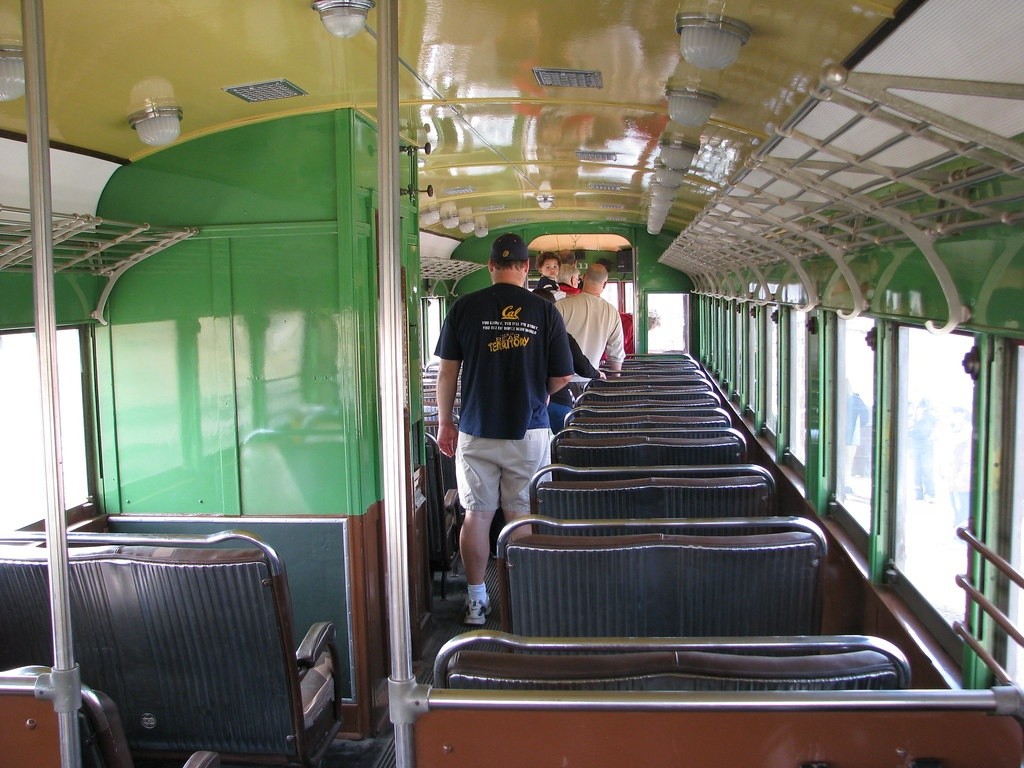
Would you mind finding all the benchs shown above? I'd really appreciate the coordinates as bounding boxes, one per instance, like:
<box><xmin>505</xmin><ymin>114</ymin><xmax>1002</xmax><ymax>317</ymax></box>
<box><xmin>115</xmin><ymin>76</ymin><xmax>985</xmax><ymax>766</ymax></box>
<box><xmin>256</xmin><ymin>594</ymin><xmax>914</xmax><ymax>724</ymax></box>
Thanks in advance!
<box><xmin>421</xmin><ymin>362</ymin><xmax>463</xmax><ymax>422</ymax></box>
<box><xmin>423</xmin><ymin>410</ymin><xmax>466</xmax><ymax>600</ymax></box>
<box><xmin>604</xmin><ymin>353</ymin><xmax>693</xmax><ymax>365</ymax></box>
<box><xmin>563</xmin><ymin>407</ymin><xmax>732</xmax><ymax>438</ymax></box>
<box><xmin>1</xmin><ymin>664</ymin><xmax>224</xmax><ymax>768</ymax></box>
<box><xmin>599</xmin><ymin>359</ymin><xmax>700</xmax><ymax>377</ymax></box>
<box><xmin>550</xmin><ymin>426</ymin><xmax>747</xmax><ymax>481</ymax></box>
<box><xmin>0</xmin><ymin>529</ymin><xmax>343</xmax><ymax>768</ymax></box>
<box><xmin>572</xmin><ymin>391</ymin><xmax>721</xmax><ymax>418</ymax></box>
<box><xmin>590</xmin><ymin>368</ymin><xmax>706</xmax><ymax>388</ymax></box>
<box><xmin>496</xmin><ymin>517</ymin><xmax>828</xmax><ymax>638</ymax></box>
<box><xmin>432</xmin><ymin>630</ymin><xmax>910</xmax><ymax>689</ymax></box>
<box><xmin>529</xmin><ymin>464</ymin><xmax>777</xmax><ymax>538</ymax></box>
<box><xmin>583</xmin><ymin>378</ymin><xmax>713</xmax><ymax>400</ymax></box>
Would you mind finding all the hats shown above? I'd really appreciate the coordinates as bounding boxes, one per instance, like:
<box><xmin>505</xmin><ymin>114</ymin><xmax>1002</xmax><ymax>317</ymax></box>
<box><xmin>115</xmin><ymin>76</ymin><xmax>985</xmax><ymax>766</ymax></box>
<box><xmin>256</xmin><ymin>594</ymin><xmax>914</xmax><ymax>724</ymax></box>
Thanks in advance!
<box><xmin>490</xmin><ymin>233</ymin><xmax>529</xmax><ymax>261</ymax></box>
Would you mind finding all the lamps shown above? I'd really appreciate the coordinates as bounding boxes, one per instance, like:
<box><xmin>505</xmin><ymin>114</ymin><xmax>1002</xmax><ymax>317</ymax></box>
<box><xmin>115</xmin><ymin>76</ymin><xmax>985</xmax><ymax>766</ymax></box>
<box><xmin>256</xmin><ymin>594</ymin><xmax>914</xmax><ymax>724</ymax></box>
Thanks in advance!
<box><xmin>646</xmin><ymin>166</ymin><xmax>689</xmax><ymax>235</ymax></box>
<box><xmin>474</xmin><ymin>225</ymin><xmax>489</xmax><ymax>237</ymax></box>
<box><xmin>459</xmin><ymin>219</ymin><xmax>475</xmax><ymax>233</ymax></box>
<box><xmin>311</xmin><ymin>0</ymin><xmax>376</xmax><ymax>39</ymax></box>
<box><xmin>0</xmin><ymin>45</ymin><xmax>25</xmax><ymax>100</ymax></box>
<box><xmin>657</xmin><ymin>141</ymin><xmax>700</xmax><ymax>170</ymax></box>
<box><xmin>663</xmin><ymin>90</ymin><xmax>720</xmax><ymax>129</ymax></box>
<box><xmin>128</xmin><ymin>105</ymin><xmax>184</xmax><ymax>148</ymax></box>
<box><xmin>440</xmin><ymin>213</ymin><xmax>459</xmax><ymax>229</ymax></box>
<box><xmin>536</xmin><ymin>196</ymin><xmax>554</xmax><ymax>209</ymax></box>
<box><xmin>419</xmin><ymin>207</ymin><xmax>440</xmax><ymax>225</ymax></box>
<box><xmin>674</xmin><ymin>11</ymin><xmax>753</xmax><ymax>71</ymax></box>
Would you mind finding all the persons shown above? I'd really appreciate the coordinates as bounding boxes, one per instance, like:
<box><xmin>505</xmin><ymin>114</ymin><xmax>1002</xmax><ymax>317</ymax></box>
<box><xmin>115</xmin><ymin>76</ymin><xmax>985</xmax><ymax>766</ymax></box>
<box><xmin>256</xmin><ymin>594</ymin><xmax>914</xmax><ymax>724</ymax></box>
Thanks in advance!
<box><xmin>434</xmin><ymin>231</ymin><xmax>575</xmax><ymax>627</ymax></box>
<box><xmin>840</xmin><ymin>379</ymin><xmax>972</xmax><ymax>503</ymax></box>
<box><xmin>532</xmin><ymin>249</ymin><xmax>662</xmax><ymax>441</ymax></box>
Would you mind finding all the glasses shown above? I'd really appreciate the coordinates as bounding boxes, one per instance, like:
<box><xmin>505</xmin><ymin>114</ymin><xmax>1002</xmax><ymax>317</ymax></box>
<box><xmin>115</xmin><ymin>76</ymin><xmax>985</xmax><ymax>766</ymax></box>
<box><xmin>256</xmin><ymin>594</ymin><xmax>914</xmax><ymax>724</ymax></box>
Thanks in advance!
<box><xmin>572</xmin><ymin>276</ymin><xmax>581</xmax><ymax>283</ymax></box>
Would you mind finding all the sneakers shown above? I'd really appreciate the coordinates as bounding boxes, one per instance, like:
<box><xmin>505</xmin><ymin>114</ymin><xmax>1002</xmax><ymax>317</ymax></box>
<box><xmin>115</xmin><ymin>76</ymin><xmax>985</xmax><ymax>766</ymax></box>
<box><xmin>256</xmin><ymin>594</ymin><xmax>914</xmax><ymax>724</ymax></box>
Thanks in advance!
<box><xmin>463</xmin><ymin>593</ymin><xmax>493</xmax><ymax>624</ymax></box>
